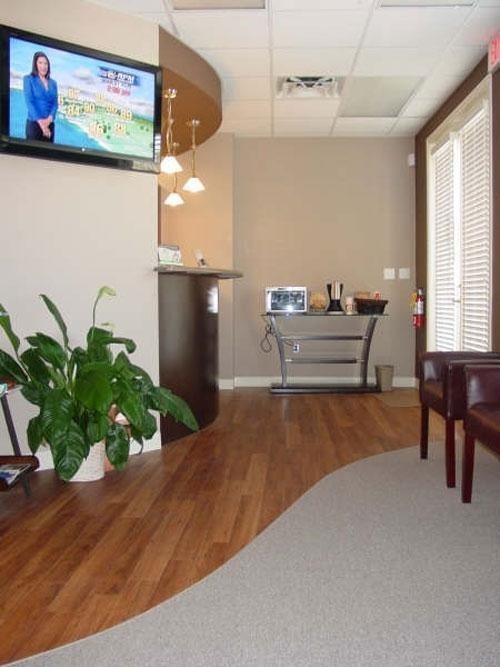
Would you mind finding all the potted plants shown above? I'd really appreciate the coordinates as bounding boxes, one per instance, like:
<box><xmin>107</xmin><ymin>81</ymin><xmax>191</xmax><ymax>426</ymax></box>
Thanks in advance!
<box><xmin>1</xmin><ymin>280</ymin><xmax>201</xmax><ymax>482</ymax></box>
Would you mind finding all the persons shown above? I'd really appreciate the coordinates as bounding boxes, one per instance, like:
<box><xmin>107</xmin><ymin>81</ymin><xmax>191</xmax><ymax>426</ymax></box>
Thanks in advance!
<box><xmin>23</xmin><ymin>51</ymin><xmax>57</xmax><ymax>143</ymax></box>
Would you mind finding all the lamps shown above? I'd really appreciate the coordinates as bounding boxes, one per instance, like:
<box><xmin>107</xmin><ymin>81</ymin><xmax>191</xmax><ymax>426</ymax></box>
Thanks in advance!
<box><xmin>160</xmin><ymin>88</ymin><xmax>206</xmax><ymax>207</ymax></box>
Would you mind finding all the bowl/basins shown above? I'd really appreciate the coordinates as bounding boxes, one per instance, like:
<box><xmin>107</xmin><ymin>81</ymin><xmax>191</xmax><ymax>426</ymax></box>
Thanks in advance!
<box><xmin>355</xmin><ymin>298</ymin><xmax>387</xmax><ymax>315</ymax></box>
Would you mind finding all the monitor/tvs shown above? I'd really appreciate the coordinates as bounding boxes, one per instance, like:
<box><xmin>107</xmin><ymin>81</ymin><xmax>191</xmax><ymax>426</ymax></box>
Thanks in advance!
<box><xmin>0</xmin><ymin>24</ymin><xmax>164</xmax><ymax>174</ymax></box>
<box><xmin>265</xmin><ymin>286</ymin><xmax>307</xmax><ymax>314</ymax></box>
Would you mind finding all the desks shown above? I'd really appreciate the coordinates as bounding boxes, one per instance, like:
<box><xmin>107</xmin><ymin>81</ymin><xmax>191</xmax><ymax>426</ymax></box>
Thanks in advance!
<box><xmin>259</xmin><ymin>313</ymin><xmax>388</xmax><ymax>394</ymax></box>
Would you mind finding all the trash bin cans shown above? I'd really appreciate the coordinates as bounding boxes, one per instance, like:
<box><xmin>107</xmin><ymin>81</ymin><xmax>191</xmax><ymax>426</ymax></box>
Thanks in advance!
<box><xmin>375</xmin><ymin>365</ymin><xmax>394</xmax><ymax>392</ymax></box>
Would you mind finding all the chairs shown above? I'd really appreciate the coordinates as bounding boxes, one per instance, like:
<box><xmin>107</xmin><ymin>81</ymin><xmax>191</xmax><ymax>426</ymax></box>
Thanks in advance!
<box><xmin>418</xmin><ymin>352</ymin><xmax>499</xmax><ymax>503</ymax></box>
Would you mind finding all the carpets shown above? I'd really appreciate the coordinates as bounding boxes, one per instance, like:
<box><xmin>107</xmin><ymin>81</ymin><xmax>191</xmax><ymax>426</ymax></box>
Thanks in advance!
<box><xmin>374</xmin><ymin>387</ymin><xmax>422</xmax><ymax>407</ymax></box>
<box><xmin>2</xmin><ymin>438</ymin><xmax>500</xmax><ymax>667</ymax></box>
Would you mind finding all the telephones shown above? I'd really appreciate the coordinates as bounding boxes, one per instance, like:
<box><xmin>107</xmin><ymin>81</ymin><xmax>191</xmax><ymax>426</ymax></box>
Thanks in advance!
<box><xmin>193</xmin><ymin>250</ymin><xmax>207</xmax><ymax>266</ymax></box>
<box><xmin>412</xmin><ymin>287</ymin><xmax>424</xmax><ymax>328</ymax></box>
